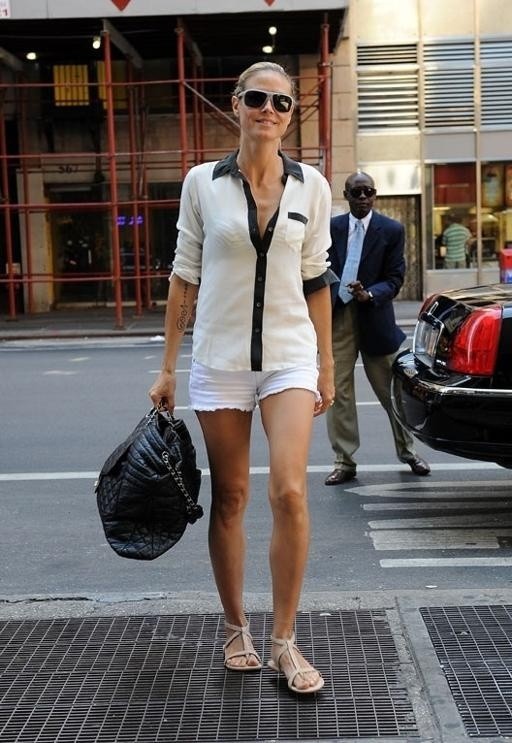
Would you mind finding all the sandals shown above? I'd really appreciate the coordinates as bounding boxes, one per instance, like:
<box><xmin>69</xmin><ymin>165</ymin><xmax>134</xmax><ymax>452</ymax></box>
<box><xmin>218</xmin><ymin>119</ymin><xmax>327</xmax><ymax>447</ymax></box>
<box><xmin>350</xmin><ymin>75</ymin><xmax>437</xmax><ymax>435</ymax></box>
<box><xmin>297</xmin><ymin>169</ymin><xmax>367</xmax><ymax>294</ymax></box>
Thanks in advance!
<box><xmin>223</xmin><ymin>620</ymin><xmax>263</xmax><ymax>671</ymax></box>
<box><xmin>267</xmin><ymin>635</ymin><xmax>325</xmax><ymax>693</ymax></box>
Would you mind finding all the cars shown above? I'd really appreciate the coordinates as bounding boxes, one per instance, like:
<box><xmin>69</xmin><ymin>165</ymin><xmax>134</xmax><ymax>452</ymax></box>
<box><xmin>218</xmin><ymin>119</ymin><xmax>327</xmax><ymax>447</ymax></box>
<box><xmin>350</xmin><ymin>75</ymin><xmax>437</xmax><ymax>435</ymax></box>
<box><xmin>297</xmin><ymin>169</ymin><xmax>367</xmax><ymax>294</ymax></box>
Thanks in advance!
<box><xmin>118</xmin><ymin>252</ymin><xmax>172</xmax><ymax>292</ymax></box>
<box><xmin>388</xmin><ymin>279</ymin><xmax>511</xmax><ymax>471</ymax></box>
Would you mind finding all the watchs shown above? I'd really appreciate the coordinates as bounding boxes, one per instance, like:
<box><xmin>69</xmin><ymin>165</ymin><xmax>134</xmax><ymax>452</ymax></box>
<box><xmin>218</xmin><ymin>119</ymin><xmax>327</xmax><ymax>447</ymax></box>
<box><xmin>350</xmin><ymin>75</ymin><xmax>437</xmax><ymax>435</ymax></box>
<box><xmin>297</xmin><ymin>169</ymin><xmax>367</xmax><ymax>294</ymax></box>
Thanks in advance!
<box><xmin>367</xmin><ymin>290</ymin><xmax>374</xmax><ymax>299</ymax></box>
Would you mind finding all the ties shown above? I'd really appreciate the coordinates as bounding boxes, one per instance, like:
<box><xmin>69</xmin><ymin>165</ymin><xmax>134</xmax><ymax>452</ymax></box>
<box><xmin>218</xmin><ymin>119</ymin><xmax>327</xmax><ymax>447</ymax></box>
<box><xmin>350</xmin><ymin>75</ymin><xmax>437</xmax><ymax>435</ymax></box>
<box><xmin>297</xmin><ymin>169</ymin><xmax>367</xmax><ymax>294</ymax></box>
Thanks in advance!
<box><xmin>337</xmin><ymin>221</ymin><xmax>365</xmax><ymax>305</ymax></box>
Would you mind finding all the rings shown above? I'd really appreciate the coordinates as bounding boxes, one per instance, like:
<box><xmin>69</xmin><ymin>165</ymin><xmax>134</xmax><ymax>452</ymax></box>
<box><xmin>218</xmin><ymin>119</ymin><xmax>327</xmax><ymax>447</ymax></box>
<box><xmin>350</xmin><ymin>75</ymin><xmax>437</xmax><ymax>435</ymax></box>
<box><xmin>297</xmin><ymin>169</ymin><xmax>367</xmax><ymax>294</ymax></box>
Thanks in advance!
<box><xmin>328</xmin><ymin>399</ymin><xmax>335</xmax><ymax>406</ymax></box>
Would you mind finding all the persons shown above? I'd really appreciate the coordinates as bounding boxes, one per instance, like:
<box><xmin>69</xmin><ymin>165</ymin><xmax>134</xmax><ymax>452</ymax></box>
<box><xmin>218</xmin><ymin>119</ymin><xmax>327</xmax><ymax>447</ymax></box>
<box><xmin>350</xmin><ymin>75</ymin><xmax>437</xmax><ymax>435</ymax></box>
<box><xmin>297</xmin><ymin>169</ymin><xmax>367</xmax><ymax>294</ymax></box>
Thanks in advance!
<box><xmin>148</xmin><ymin>62</ymin><xmax>340</xmax><ymax>698</ymax></box>
<box><xmin>323</xmin><ymin>170</ymin><xmax>432</xmax><ymax>485</ymax></box>
<box><xmin>440</xmin><ymin>212</ymin><xmax>472</xmax><ymax>269</ymax></box>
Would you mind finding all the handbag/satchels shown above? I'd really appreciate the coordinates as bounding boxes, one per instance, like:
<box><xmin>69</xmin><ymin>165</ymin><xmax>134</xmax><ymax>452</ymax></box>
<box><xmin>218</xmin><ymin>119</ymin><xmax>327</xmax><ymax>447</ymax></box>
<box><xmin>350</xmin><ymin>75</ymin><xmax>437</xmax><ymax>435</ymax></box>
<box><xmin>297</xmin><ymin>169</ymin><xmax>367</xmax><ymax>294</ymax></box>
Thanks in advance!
<box><xmin>95</xmin><ymin>400</ymin><xmax>204</xmax><ymax>561</ymax></box>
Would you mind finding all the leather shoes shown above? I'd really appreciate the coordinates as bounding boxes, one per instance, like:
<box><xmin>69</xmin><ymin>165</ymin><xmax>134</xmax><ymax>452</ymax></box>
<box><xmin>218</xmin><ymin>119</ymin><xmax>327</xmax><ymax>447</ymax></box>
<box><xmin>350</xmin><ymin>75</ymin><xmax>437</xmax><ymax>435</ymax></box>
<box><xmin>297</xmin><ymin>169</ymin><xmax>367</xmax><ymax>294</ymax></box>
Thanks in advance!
<box><xmin>407</xmin><ymin>455</ymin><xmax>430</xmax><ymax>475</ymax></box>
<box><xmin>325</xmin><ymin>468</ymin><xmax>355</xmax><ymax>485</ymax></box>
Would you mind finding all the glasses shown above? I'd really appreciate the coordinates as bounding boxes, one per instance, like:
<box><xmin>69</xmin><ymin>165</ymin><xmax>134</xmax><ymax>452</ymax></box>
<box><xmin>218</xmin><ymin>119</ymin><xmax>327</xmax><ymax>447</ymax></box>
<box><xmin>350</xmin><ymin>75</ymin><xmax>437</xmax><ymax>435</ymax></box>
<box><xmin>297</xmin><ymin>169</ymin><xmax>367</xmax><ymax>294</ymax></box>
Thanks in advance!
<box><xmin>349</xmin><ymin>187</ymin><xmax>376</xmax><ymax>198</ymax></box>
<box><xmin>238</xmin><ymin>89</ymin><xmax>294</xmax><ymax>113</ymax></box>
